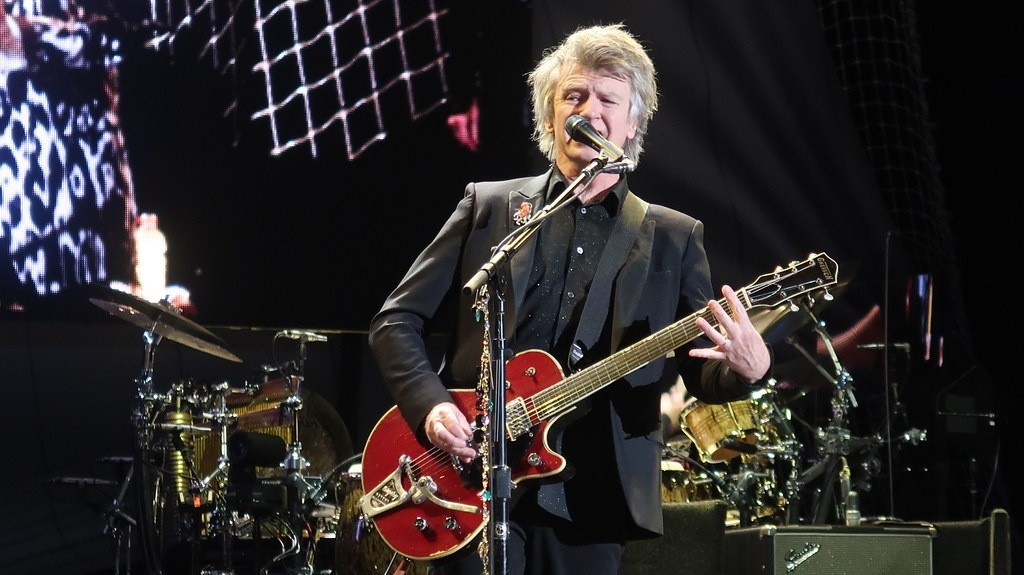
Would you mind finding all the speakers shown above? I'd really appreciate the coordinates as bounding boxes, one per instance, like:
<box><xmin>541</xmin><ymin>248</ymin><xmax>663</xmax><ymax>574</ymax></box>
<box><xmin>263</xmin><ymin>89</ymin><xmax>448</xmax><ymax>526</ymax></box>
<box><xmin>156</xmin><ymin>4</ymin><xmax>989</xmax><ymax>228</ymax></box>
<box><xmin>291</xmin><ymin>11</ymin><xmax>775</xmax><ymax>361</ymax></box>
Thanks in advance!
<box><xmin>758</xmin><ymin>520</ymin><xmax>993</xmax><ymax>575</ymax></box>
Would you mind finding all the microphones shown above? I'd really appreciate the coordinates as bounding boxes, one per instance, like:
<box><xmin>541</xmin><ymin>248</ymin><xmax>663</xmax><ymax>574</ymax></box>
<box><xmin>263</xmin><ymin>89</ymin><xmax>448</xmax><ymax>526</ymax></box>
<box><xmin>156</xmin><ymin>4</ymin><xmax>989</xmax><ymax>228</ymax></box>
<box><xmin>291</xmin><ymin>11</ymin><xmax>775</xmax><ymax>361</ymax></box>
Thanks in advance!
<box><xmin>49</xmin><ymin>476</ymin><xmax>119</xmax><ymax>487</ymax></box>
<box><xmin>564</xmin><ymin>114</ymin><xmax>636</xmax><ymax>174</ymax></box>
<box><xmin>155</xmin><ymin>423</ymin><xmax>212</xmax><ymax>434</ymax></box>
<box><xmin>282</xmin><ymin>329</ymin><xmax>327</xmax><ymax>342</ymax></box>
<box><xmin>97</xmin><ymin>457</ymin><xmax>134</xmax><ymax>464</ymax></box>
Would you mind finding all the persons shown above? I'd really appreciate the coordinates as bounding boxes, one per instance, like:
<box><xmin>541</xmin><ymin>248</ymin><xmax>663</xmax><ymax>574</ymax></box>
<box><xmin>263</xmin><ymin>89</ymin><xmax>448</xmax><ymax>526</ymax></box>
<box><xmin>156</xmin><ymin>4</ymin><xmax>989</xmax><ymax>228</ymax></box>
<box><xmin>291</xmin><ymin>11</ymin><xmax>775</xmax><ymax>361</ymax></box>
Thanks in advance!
<box><xmin>368</xmin><ymin>23</ymin><xmax>772</xmax><ymax>573</ymax></box>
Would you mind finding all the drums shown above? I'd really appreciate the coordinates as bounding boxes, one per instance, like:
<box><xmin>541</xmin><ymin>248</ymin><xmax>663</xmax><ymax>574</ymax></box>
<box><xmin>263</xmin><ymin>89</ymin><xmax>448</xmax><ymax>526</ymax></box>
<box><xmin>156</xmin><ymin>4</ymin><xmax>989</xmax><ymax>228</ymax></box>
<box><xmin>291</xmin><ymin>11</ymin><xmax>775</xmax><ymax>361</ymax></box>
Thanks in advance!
<box><xmin>333</xmin><ymin>464</ymin><xmax>430</xmax><ymax>574</ymax></box>
<box><xmin>660</xmin><ymin>458</ymin><xmax>696</xmax><ymax>504</ymax></box>
<box><xmin>678</xmin><ymin>389</ymin><xmax>766</xmax><ymax>466</ymax></box>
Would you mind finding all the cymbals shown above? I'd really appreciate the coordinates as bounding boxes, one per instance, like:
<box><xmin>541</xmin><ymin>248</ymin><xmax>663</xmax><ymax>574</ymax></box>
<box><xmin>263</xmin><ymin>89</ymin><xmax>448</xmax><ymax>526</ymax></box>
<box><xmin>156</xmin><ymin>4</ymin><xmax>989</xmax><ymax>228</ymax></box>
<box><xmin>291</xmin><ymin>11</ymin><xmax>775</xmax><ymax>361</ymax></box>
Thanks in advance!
<box><xmin>83</xmin><ymin>278</ymin><xmax>251</xmax><ymax>368</ymax></box>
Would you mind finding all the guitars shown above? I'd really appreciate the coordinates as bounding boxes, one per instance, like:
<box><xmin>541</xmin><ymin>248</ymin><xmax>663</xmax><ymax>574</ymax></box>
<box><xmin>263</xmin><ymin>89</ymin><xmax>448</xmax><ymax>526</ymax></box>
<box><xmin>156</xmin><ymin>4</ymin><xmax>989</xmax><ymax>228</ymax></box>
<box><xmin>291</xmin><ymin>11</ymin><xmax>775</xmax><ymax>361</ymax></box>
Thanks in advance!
<box><xmin>358</xmin><ymin>249</ymin><xmax>839</xmax><ymax>567</ymax></box>
